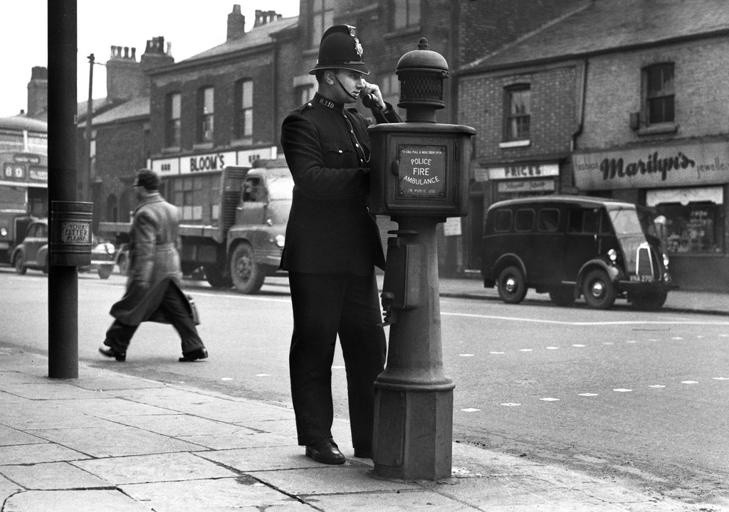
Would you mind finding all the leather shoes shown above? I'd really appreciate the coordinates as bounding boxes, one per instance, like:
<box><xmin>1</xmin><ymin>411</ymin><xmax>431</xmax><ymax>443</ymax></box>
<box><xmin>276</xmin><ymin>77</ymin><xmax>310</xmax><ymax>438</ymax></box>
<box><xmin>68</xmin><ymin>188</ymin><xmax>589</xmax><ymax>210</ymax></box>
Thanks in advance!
<box><xmin>306</xmin><ymin>438</ymin><xmax>373</xmax><ymax>465</ymax></box>
<box><xmin>179</xmin><ymin>347</ymin><xmax>208</xmax><ymax>361</ymax></box>
<box><xmin>99</xmin><ymin>348</ymin><xmax>126</xmax><ymax>361</ymax></box>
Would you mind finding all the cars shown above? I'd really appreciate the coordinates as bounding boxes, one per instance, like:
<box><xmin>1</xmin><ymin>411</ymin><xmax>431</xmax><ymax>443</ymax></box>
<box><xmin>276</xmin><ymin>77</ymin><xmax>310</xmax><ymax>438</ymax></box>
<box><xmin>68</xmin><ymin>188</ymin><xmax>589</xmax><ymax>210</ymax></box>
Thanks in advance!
<box><xmin>11</xmin><ymin>219</ymin><xmax>116</xmax><ymax>278</ymax></box>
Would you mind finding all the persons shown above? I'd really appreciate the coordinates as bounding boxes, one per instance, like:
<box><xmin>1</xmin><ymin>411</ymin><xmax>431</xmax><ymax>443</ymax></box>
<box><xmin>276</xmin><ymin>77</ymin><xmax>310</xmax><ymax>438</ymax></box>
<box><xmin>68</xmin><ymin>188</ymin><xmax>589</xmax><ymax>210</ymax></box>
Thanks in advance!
<box><xmin>277</xmin><ymin>25</ymin><xmax>402</xmax><ymax>464</ymax></box>
<box><xmin>98</xmin><ymin>168</ymin><xmax>208</xmax><ymax>362</ymax></box>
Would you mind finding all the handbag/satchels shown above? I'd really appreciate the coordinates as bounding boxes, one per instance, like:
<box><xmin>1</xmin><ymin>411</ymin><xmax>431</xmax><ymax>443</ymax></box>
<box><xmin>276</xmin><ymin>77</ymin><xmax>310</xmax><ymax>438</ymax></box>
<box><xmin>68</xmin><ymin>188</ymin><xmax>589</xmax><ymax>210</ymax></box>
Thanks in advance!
<box><xmin>187</xmin><ymin>295</ymin><xmax>201</xmax><ymax>325</ymax></box>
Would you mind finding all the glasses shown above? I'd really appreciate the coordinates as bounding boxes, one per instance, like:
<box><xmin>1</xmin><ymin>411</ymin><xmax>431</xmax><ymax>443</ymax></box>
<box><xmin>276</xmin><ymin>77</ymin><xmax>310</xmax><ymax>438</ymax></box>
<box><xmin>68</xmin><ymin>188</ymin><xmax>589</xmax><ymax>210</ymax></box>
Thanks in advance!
<box><xmin>131</xmin><ymin>185</ymin><xmax>144</xmax><ymax>188</ymax></box>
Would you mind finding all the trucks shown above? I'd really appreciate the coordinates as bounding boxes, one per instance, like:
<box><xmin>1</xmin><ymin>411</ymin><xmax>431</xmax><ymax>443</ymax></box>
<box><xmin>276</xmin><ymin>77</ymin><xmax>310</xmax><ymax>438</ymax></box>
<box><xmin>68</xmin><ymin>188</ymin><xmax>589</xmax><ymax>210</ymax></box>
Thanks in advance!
<box><xmin>98</xmin><ymin>160</ymin><xmax>295</xmax><ymax>294</ymax></box>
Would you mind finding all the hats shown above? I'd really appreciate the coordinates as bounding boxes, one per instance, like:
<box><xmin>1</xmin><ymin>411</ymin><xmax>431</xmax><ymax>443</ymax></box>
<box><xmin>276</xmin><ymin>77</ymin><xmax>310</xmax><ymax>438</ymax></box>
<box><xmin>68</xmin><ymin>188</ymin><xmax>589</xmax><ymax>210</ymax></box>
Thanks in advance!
<box><xmin>309</xmin><ymin>24</ymin><xmax>368</xmax><ymax>75</ymax></box>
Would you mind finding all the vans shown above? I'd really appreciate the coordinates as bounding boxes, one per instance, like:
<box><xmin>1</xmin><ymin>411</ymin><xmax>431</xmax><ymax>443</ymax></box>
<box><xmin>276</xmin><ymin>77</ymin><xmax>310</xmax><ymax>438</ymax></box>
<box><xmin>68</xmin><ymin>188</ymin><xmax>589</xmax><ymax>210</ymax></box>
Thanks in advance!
<box><xmin>482</xmin><ymin>195</ymin><xmax>672</xmax><ymax>309</ymax></box>
